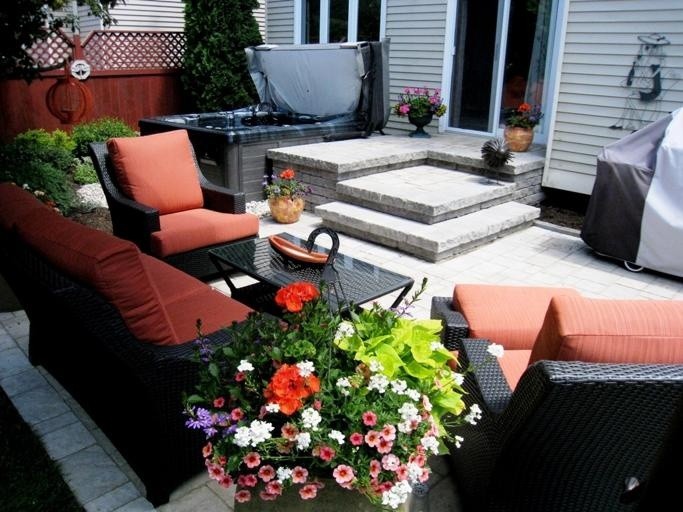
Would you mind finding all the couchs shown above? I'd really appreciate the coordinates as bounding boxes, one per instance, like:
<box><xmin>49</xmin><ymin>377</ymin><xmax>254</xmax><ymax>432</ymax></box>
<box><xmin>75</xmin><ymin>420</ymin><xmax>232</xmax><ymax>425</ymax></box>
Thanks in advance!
<box><xmin>0</xmin><ymin>181</ymin><xmax>261</xmax><ymax>507</ymax></box>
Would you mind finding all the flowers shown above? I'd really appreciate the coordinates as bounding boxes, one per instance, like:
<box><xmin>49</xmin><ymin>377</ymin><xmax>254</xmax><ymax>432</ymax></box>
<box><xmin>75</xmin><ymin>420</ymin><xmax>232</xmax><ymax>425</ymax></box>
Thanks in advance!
<box><xmin>502</xmin><ymin>102</ymin><xmax>545</xmax><ymax>130</ymax></box>
<box><xmin>260</xmin><ymin>168</ymin><xmax>314</xmax><ymax>202</ymax></box>
<box><xmin>390</xmin><ymin>86</ymin><xmax>447</xmax><ymax>118</ymax></box>
<box><xmin>177</xmin><ymin>274</ymin><xmax>507</xmax><ymax>511</ymax></box>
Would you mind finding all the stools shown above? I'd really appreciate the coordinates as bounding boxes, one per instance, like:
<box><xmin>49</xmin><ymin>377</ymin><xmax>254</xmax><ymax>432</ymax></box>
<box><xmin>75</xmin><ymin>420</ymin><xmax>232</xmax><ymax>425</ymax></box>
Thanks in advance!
<box><xmin>430</xmin><ymin>283</ymin><xmax>578</xmax><ymax>351</ymax></box>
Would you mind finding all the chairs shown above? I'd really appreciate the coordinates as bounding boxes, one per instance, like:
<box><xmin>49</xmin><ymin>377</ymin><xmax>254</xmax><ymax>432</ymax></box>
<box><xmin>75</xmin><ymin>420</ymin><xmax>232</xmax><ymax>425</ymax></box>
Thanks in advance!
<box><xmin>85</xmin><ymin>129</ymin><xmax>259</xmax><ymax>281</ymax></box>
<box><xmin>441</xmin><ymin>294</ymin><xmax>683</xmax><ymax>512</ymax></box>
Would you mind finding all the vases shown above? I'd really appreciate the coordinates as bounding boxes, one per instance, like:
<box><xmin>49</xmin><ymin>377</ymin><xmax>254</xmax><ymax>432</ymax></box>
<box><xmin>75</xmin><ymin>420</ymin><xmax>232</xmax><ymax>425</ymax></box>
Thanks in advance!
<box><xmin>268</xmin><ymin>197</ymin><xmax>304</xmax><ymax>224</ymax></box>
<box><xmin>408</xmin><ymin>112</ymin><xmax>432</xmax><ymax>138</ymax></box>
<box><xmin>232</xmin><ymin>476</ymin><xmax>409</xmax><ymax>511</ymax></box>
<box><xmin>504</xmin><ymin>126</ymin><xmax>533</xmax><ymax>153</ymax></box>
